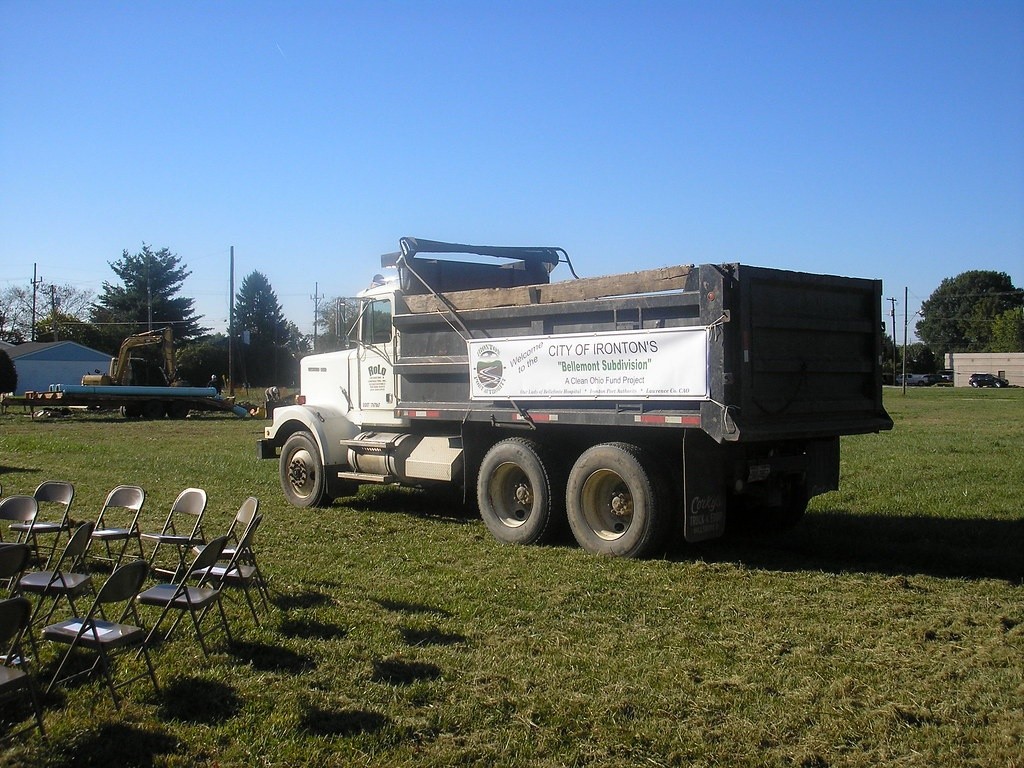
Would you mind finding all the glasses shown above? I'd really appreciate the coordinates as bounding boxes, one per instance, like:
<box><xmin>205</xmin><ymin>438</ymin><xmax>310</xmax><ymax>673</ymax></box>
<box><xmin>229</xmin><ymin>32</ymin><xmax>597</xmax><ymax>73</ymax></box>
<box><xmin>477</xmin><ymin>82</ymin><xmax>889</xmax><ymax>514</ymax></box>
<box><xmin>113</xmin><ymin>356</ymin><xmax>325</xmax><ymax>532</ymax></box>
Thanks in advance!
<box><xmin>214</xmin><ymin>378</ymin><xmax>217</xmax><ymax>379</ymax></box>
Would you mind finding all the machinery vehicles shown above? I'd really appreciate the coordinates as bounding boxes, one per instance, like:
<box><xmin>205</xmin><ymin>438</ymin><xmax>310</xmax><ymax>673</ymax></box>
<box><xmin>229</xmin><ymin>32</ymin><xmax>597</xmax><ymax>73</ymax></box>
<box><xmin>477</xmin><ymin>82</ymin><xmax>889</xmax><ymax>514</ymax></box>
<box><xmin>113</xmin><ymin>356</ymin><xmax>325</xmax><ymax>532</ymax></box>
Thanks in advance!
<box><xmin>79</xmin><ymin>326</ymin><xmax>180</xmax><ymax>387</ymax></box>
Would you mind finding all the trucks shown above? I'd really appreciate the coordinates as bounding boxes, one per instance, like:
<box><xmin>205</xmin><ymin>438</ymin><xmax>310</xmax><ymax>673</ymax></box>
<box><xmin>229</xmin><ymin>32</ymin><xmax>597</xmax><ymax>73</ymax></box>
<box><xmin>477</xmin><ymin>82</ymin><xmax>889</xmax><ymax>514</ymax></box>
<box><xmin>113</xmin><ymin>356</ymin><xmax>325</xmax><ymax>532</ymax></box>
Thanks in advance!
<box><xmin>257</xmin><ymin>235</ymin><xmax>897</xmax><ymax>559</ymax></box>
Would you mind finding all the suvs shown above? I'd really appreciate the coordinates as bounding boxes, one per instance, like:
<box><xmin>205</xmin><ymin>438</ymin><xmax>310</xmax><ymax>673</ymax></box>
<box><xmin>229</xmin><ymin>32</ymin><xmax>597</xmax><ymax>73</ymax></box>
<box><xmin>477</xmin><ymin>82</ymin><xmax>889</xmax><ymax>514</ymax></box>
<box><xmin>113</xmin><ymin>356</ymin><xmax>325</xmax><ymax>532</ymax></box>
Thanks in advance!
<box><xmin>968</xmin><ymin>373</ymin><xmax>1010</xmax><ymax>389</ymax></box>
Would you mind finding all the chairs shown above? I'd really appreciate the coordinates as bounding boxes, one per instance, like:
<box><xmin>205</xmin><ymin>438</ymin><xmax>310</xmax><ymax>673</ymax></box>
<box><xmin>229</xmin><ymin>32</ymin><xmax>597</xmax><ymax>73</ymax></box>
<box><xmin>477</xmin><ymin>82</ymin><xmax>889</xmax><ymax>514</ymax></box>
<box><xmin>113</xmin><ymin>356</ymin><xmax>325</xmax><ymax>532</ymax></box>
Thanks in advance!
<box><xmin>138</xmin><ymin>488</ymin><xmax>216</xmax><ymax>587</ymax></box>
<box><xmin>188</xmin><ymin>514</ymin><xmax>272</xmax><ymax>632</ymax></box>
<box><xmin>191</xmin><ymin>497</ymin><xmax>265</xmax><ymax>609</ymax></box>
<box><xmin>39</xmin><ymin>557</ymin><xmax>164</xmax><ymax>729</ymax></box>
<box><xmin>0</xmin><ymin>596</ymin><xmax>54</xmax><ymax>759</ymax></box>
<box><xmin>0</xmin><ymin>544</ymin><xmax>36</xmax><ymax>643</ymax></box>
<box><xmin>18</xmin><ymin>520</ymin><xmax>110</xmax><ymax>645</ymax></box>
<box><xmin>0</xmin><ymin>493</ymin><xmax>41</xmax><ymax>594</ymax></box>
<box><xmin>77</xmin><ymin>484</ymin><xmax>149</xmax><ymax>574</ymax></box>
<box><xmin>134</xmin><ymin>534</ymin><xmax>235</xmax><ymax>664</ymax></box>
<box><xmin>7</xmin><ymin>479</ymin><xmax>81</xmax><ymax>572</ymax></box>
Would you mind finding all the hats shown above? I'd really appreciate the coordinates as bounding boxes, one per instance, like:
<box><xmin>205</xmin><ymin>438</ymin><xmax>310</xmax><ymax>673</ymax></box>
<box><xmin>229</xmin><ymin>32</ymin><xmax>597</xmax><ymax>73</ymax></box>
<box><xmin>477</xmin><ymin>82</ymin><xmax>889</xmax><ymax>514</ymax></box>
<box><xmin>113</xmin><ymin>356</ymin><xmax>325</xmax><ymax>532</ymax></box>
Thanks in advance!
<box><xmin>273</xmin><ymin>387</ymin><xmax>279</xmax><ymax>393</ymax></box>
<box><xmin>211</xmin><ymin>375</ymin><xmax>217</xmax><ymax>378</ymax></box>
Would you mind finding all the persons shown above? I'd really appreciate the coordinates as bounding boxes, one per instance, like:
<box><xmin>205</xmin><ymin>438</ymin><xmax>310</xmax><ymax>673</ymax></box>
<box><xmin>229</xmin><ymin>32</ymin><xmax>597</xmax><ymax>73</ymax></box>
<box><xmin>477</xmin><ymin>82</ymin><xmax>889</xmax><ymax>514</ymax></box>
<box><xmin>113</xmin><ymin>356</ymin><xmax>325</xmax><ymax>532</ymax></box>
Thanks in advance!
<box><xmin>265</xmin><ymin>386</ymin><xmax>280</xmax><ymax>402</ymax></box>
<box><xmin>208</xmin><ymin>375</ymin><xmax>222</xmax><ymax>396</ymax></box>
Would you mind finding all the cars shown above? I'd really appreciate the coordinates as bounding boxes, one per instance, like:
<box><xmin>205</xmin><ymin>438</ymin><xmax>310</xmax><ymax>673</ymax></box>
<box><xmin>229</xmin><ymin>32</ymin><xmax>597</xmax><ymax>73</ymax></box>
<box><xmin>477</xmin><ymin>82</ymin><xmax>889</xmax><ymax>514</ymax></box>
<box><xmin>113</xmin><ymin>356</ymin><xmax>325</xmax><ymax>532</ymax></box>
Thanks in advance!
<box><xmin>895</xmin><ymin>371</ymin><xmax>954</xmax><ymax>387</ymax></box>
